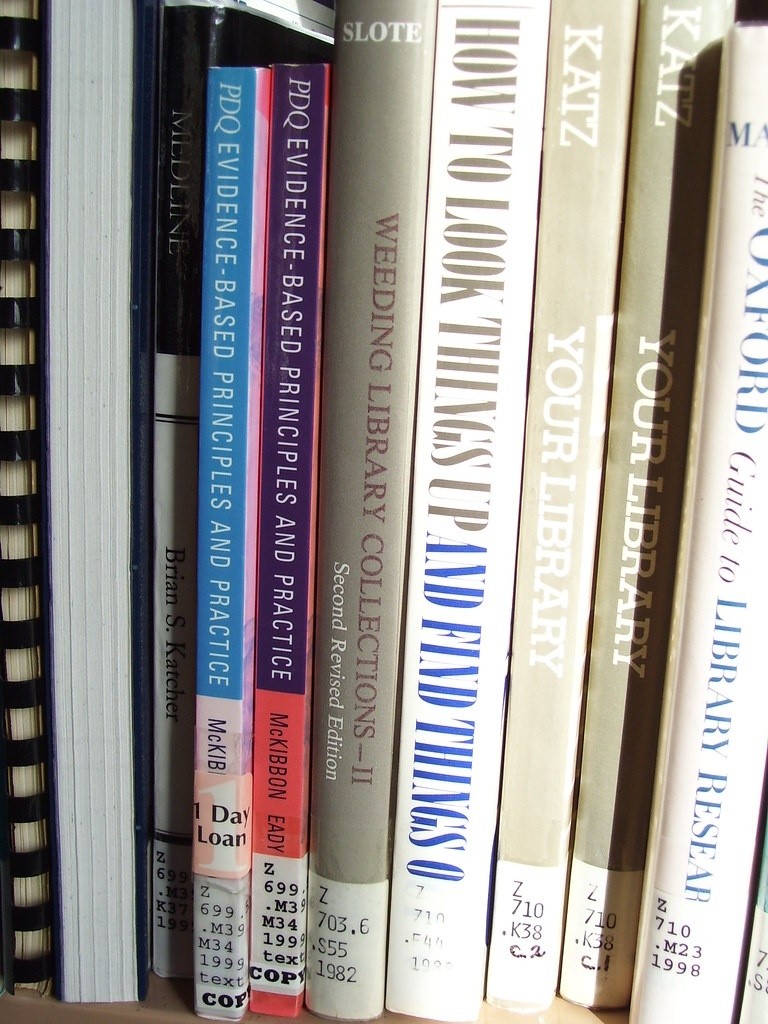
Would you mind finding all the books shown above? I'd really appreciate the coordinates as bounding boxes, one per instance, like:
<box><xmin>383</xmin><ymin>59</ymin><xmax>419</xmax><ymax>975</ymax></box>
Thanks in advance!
<box><xmin>0</xmin><ymin>0</ymin><xmax>767</xmax><ymax>1022</ymax></box>
